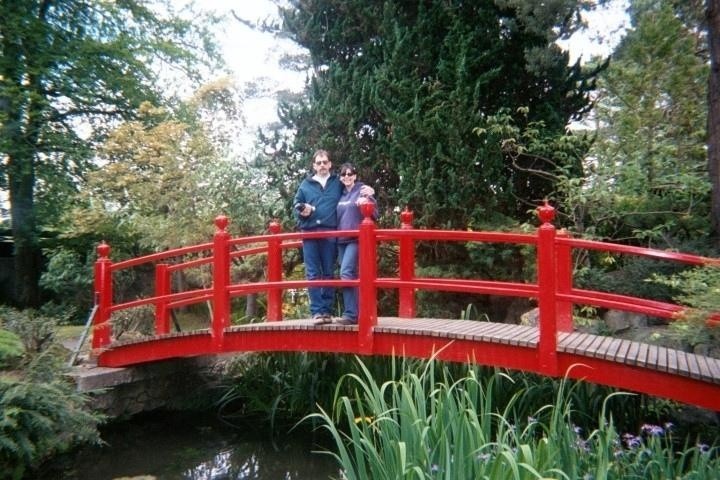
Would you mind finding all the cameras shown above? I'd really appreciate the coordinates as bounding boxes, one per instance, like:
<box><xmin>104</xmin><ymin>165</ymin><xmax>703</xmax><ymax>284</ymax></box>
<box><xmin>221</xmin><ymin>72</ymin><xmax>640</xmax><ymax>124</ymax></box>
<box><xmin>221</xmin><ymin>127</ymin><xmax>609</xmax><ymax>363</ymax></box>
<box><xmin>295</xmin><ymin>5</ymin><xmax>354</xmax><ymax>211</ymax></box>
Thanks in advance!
<box><xmin>295</xmin><ymin>203</ymin><xmax>315</xmax><ymax>216</ymax></box>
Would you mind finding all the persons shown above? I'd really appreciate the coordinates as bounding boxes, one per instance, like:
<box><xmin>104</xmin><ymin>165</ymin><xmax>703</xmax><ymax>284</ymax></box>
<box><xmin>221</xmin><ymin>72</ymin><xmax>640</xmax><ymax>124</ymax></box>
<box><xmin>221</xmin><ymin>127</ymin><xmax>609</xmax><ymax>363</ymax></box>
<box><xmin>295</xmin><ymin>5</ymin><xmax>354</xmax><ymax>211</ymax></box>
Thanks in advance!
<box><xmin>336</xmin><ymin>162</ymin><xmax>379</xmax><ymax>325</ymax></box>
<box><xmin>292</xmin><ymin>149</ymin><xmax>341</xmax><ymax>325</ymax></box>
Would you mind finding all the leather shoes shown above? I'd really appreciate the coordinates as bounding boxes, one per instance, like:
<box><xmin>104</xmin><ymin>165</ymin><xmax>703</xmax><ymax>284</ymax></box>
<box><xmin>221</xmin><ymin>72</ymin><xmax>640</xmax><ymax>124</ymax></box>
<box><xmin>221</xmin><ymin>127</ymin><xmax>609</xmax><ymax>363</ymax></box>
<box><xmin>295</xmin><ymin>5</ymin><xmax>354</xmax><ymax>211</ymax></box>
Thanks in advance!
<box><xmin>313</xmin><ymin>313</ymin><xmax>353</xmax><ymax>325</ymax></box>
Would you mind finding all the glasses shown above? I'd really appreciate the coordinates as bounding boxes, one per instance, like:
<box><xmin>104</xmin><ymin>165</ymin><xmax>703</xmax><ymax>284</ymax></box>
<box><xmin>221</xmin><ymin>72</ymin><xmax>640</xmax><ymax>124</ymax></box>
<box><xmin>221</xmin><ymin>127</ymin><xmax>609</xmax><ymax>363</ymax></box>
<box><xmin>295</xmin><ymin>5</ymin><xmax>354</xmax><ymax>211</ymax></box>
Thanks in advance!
<box><xmin>340</xmin><ymin>173</ymin><xmax>354</xmax><ymax>176</ymax></box>
<box><xmin>316</xmin><ymin>160</ymin><xmax>328</xmax><ymax>165</ymax></box>
